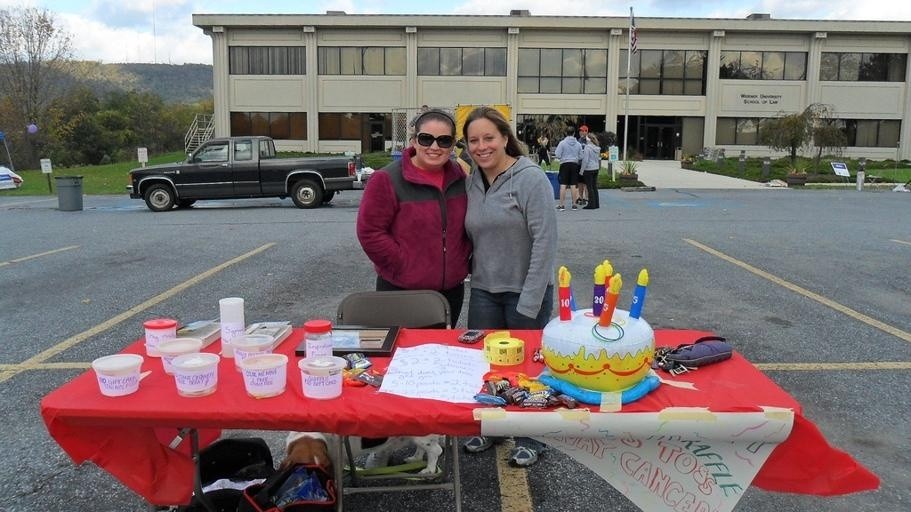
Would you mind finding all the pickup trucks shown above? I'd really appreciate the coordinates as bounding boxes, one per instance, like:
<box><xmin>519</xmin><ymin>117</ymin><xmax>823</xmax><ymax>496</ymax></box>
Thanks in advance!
<box><xmin>126</xmin><ymin>137</ymin><xmax>358</xmax><ymax>211</ymax></box>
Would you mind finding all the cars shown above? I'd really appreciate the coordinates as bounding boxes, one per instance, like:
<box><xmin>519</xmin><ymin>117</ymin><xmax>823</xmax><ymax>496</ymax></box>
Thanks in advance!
<box><xmin>0</xmin><ymin>167</ymin><xmax>23</xmax><ymax>189</ymax></box>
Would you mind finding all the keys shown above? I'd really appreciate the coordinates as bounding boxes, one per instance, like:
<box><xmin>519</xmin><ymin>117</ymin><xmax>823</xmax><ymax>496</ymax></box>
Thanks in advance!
<box><xmin>662</xmin><ymin>357</ymin><xmax>677</xmax><ymax>377</ymax></box>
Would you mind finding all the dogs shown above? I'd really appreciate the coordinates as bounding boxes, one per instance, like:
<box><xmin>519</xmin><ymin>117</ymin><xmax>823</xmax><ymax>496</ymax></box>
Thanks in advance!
<box><xmin>361</xmin><ymin>435</ymin><xmax>442</xmax><ymax>481</ymax></box>
<box><xmin>282</xmin><ymin>432</ymin><xmax>332</xmax><ymax>475</ymax></box>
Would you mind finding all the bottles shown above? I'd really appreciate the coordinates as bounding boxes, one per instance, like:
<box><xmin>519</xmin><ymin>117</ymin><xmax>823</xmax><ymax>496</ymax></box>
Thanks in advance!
<box><xmin>304</xmin><ymin>321</ymin><xmax>334</xmax><ymax>360</ymax></box>
<box><xmin>144</xmin><ymin>320</ymin><xmax>177</xmax><ymax>357</ymax></box>
<box><xmin>219</xmin><ymin>298</ymin><xmax>244</xmax><ymax>357</ymax></box>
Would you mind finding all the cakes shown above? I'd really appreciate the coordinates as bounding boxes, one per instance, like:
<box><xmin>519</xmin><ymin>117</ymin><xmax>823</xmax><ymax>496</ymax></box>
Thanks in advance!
<box><xmin>539</xmin><ymin>261</ymin><xmax>659</xmax><ymax>405</ymax></box>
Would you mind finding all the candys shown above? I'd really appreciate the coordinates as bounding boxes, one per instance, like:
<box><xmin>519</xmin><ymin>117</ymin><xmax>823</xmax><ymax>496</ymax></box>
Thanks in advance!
<box><xmin>502</xmin><ymin>374</ymin><xmax>554</xmax><ymax>405</ymax></box>
<box><xmin>557</xmin><ymin>392</ymin><xmax>578</xmax><ymax>408</ymax></box>
<box><xmin>352</xmin><ymin>358</ymin><xmax>372</xmax><ymax>372</ymax></box>
<box><xmin>522</xmin><ymin>397</ymin><xmax>548</xmax><ymax>408</ymax></box>
<box><xmin>340</xmin><ymin>378</ymin><xmax>361</xmax><ymax>388</ymax></box>
<box><xmin>343</xmin><ymin>352</ymin><xmax>364</xmax><ymax>378</ymax></box>
<box><xmin>486</xmin><ymin>377</ymin><xmax>512</xmax><ymax>395</ymax></box>
<box><xmin>534</xmin><ymin>348</ymin><xmax>545</xmax><ymax>364</ymax></box>
<box><xmin>474</xmin><ymin>394</ymin><xmax>506</xmax><ymax>405</ymax></box>
<box><xmin>357</xmin><ymin>372</ymin><xmax>384</xmax><ymax>388</ymax></box>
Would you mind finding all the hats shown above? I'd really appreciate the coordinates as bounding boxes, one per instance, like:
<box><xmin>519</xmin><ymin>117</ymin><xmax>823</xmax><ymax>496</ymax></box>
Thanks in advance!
<box><xmin>578</xmin><ymin>125</ymin><xmax>589</xmax><ymax>131</ymax></box>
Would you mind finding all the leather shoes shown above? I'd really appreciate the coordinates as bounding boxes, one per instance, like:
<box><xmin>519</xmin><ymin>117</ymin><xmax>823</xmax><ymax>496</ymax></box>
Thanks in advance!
<box><xmin>583</xmin><ymin>206</ymin><xmax>594</xmax><ymax>209</ymax></box>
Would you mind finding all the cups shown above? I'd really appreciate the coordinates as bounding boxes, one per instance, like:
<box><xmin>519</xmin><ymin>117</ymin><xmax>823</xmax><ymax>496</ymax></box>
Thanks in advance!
<box><xmin>218</xmin><ymin>297</ymin><xmax>247</xmax><ymax>357</ymax></box>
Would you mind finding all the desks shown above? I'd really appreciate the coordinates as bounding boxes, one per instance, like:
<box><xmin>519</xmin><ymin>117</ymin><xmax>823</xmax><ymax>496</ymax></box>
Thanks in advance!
<box><xmin>42</xmin><ymin>327</ymin><xmax>794</xmax><ymax>512</ymax></box>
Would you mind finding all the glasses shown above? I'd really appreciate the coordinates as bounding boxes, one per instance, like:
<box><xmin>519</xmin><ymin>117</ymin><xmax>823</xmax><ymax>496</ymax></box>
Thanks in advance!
<box><xmin>416</xmin><ymin>133</ymin><xmax>454</xmax><ymax>148</ymax></box>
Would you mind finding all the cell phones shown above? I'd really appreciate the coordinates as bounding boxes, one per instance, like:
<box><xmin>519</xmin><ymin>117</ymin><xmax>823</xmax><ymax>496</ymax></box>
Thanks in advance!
<box><xmin>459</xmin><ymin>330</ymin><xmax>484</xmax><ymax>347</ymax></box>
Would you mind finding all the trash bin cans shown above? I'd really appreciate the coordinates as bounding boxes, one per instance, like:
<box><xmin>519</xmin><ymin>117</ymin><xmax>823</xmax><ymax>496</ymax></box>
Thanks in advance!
<box><xmin>54</xmin><ymin>176</ymin><xmax>83</xmax><ymax>211</ymax></box>
<box><xmin>545</xmin><ymin>171</ymin><xmax>560</xmax><ymax>200</ymax></box>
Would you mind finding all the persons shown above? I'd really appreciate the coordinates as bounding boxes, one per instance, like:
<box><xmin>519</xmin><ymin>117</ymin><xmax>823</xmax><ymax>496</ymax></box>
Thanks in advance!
<box><xmin>535</xmin><ymin>130</ymin><xmax>551</xmax><ymax>166</ymax></box>
<box><xmin>575</xmin><ymin>126</ymin><xmax>590</xmax><ymax>205</ymax></box>
<box><xmin>463</xmin><ymin>109</ymin><xmax>557</xmax><ymax>467</ymax></box>
<box><xmin>580</xmin><ymin>133</ymin><xmax>601</xmax><ymax>210</ymax></box>
<box><xmin>555</xmin><ymin>125</ymin><xmax>582</xmax><ymax>211</ymax></box>
<box><xmin>357</xmin><ymin>109</ymin><xmax>466</xmax><ymax>470</ymax></box>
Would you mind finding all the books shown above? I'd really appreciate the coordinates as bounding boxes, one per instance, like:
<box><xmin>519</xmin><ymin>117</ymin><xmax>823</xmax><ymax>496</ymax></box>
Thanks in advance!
<box><xmin>177</xmin><ymin>316</ymin><xmax>221</xmax><ymax>349</ymax></box>
<box><xmin>245</xmin><ymin>321</ymin><xmax>294</xmax><ymax>348</ymax></box>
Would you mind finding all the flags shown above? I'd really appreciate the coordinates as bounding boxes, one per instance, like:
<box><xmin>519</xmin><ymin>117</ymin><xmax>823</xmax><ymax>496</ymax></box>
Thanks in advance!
<box><xmin>628</xmin><ymin>8</ymin><xmax>637</xmax><ymax>53</ymax></box>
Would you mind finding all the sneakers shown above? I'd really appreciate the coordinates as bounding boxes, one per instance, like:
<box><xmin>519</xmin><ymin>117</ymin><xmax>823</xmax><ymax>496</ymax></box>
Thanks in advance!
<box><xmin>462</xmin><ymin>436</ymin><xmax>503</xmax><ymax>452</ymax></box>
<box><xmin>555</xmin><ymin>205</ymin><xmax>565</xmax><ymax>211</ymax></box>
<box><xmin>571</xmin><ymin>205</ymin><xmax>578</xmax><ymax>210</ymax></box>
<box><xmin>576</xmin><ymin>198</ymin><xmax>582</xmax><ymax>204</ymax></box>
<box><xmin>365</xmin><ymin>449</ymin><xmax>391</xmax><ymax>469</ymax></box>
<box><xmin>582</xmin><ymin>199</ymin><xmax>588</xmax><ymax>208</ymax></box>
<box><xmin>508</xmin><ymin>444</ymin><xmax>546</xmax><ymax>468</ymax></box>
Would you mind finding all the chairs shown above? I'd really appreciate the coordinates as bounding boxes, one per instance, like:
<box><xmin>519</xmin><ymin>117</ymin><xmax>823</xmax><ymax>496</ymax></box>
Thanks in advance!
<box><xmin>336</xmin><ymin>292</ymin><xmax>462</xmax><ymax>512</ymax></box>
